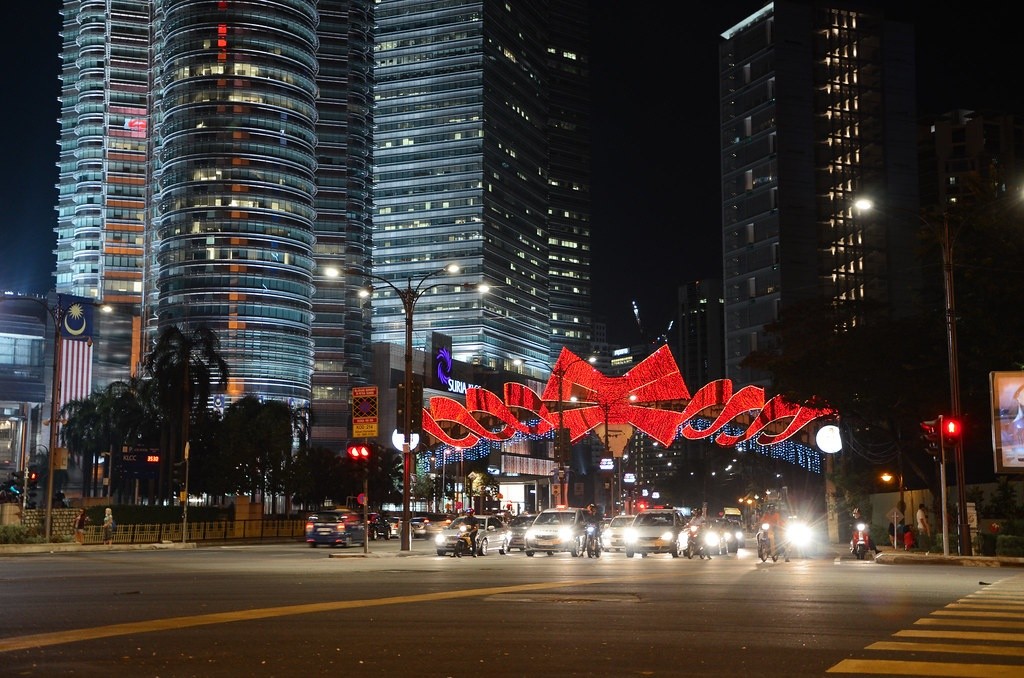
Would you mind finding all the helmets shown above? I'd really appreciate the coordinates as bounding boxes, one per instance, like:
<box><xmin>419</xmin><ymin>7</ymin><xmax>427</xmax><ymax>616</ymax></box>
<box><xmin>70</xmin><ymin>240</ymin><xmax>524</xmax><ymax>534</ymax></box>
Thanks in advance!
<box><xmin>693</xmin><ymin>508</ymin><xmax>703</xmax><ymax>515</ymax></box>
<box><xmin>853</xmin><ymin>508</ymin><xmax>861</xmax><ymax>515</ymax></box>
<box><xmin>767</xmin><ymin>503</ymin><xmax>776</xmax><ymax>512</ymax></box>
<box><xmin>587</xmin><ymin>504</ymin><xmax>596</xmax><ymax>511</ymax></box>
<box><xmin>466</xmin><ymin>508</ymin><xmax>475</xmax><ymax>513</ymax></box>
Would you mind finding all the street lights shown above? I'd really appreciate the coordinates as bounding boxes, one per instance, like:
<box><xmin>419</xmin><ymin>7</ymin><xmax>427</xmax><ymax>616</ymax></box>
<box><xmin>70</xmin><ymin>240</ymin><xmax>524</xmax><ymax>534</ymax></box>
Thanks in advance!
<box><xmin>880</xmin><ymin>470</ymin><xmax>906</xmax><ymax>524</ymax></box>
<box><xmin>324</xmin><ymin>259</ymin><xmax>490</xmax><ymax>552</ymax></box>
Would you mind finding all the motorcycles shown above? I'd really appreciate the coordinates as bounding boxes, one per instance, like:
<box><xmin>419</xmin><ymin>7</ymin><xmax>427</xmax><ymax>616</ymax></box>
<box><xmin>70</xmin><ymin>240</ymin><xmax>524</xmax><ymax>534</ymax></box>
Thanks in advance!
<box><xmin>849</xmin><ymin>521</ymin><xmax>872</xmax><ymax>559</ymax></box>
<box><xmin>756</xmin><ymin>518</ymin><xmax>780</xmax><ymax>563</ymax></box>
<box><xmin>684</xmin><ymin>522</ymin><xmax>706</xmax><ymax>560</ymax></box>
<box><xmin>580</xmin><ymin>521</ymin><xmax>604</xmax><ymax>558</ymax></box>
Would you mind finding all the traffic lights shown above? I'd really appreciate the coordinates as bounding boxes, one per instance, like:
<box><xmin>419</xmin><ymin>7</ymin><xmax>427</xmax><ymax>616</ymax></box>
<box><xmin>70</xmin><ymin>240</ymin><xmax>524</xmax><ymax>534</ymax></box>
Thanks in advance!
<box><xmin>345</xmin><ymin>444</ymin><xmax>371</xmax><ymax>460</ymax></box>
<box><xmin>920</xmin><ymin>414</ymin><xmax>943</xmax><ymax>463</ymax></box>
<box><xmin>944</xmin><ymin>415</ymin><xmax>961</xmax><ymax>448</ymax></box>
<box><xmin>27</xmin><ymin>469</ymin><xmax>40</xmax><ymax>510</ymax></box>
<box><xmin>9</xmin><ymin>471</ymin><xmax>25</xmax><ymax>494</ymax></box>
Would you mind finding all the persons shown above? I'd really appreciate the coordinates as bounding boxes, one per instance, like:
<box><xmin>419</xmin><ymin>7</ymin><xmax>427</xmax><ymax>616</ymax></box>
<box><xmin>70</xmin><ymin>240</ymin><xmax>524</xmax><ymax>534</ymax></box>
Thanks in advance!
<box><xmin>579</xmin><ymin>504</ymin><xmax>603</xmax><ymax>558</ymax></box>
<box><xmin>455</xmin><ymin>508</ymin><xmax>479</xmax><ymax>557</ymax></box>
<box><xmin>889</xmin><ymin>503</ymin><xmax>930</xmax><ymax>551</ymax></box>
<box><xmin>74</xmin><ymin>509</ymin><xmax>85</xmax><ymax>544</ymax></box>
<box><xmin>685</xmin><ymin>508</ymin><xmax>711</xmax><ymax>560</ymax></box>
<box><xmin>850</xmin><ymin>508</ymin><xmax>881</xmax><ymax>554</ymax></box>
<box><xmin>104</xmin><ymin>508</ymin><xmax>113</xmax><ymax>546</ymax></box>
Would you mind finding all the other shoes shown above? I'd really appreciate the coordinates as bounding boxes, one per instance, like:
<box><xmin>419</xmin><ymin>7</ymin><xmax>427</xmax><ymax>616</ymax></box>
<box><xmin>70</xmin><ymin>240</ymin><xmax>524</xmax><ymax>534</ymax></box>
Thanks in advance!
<box><xmin>450</xmin><ymin>552</ymin><xmax>455</xmax><ymax>557</ymax></box>
<box><xmin>707</xmin><ymin>555</ymin><xmax>711</xmax><ymax>559</ymax></box>
<box><xmin>473</xmin><ymin>553</ymin><xmax>477</xmax><ymax>557</ymax></box>
<box><xmin>785</xmin><ymin>558</ymin><xmax>790</xmax><ymax>562</ymax></box>
<box><xmin>578</xmin><ymin>553</ymin><xmax>584</xmax><ymax>557</ymax></box>
<box><xmin>876</xmin><ymin>550</ymin><xmax>882</xmax><ymax>554</ymax></box>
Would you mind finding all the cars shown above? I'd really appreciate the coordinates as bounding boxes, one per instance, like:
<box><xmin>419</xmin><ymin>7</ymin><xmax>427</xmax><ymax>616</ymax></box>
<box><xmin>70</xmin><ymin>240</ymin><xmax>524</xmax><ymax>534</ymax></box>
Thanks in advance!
<box><xmin>357</xmin><ymin>503</ymin><xmax>748</xmax><ymax>557</ymax></box>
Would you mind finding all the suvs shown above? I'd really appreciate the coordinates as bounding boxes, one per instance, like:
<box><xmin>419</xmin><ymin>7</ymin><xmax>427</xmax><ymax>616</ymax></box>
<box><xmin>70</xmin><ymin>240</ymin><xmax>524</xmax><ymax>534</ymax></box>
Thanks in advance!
<box><xmin>305</xmin><ymin>508</ymin><xmax>365</xmax><ymax>548</ymax></box>
